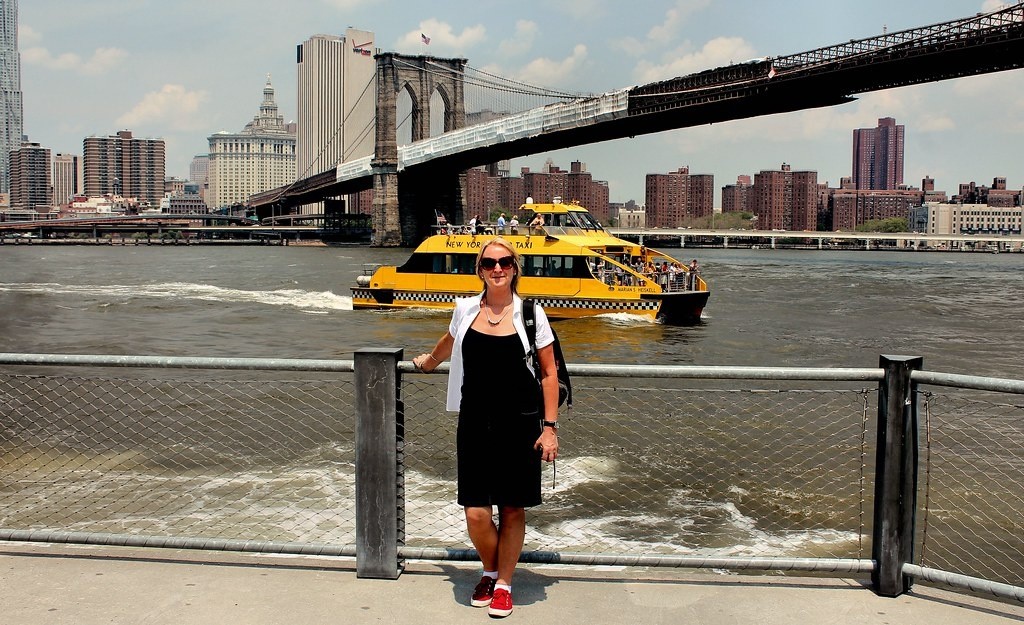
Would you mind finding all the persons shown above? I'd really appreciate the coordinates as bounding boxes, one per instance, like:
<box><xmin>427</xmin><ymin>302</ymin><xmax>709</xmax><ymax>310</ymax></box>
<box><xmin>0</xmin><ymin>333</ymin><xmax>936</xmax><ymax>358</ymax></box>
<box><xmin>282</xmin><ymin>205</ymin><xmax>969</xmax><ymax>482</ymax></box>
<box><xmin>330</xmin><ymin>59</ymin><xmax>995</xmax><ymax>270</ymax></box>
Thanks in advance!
<box><xmin>413</xmin><ymin>237</ymin><xmax>559</xmax><ymax>616</ymax></box>
<box><xmin>530</xmin><ymin>212</ymin><xmax>545</xmax><ymax>235</ymax></box>
<box><xmin>688</xmin><ymin>259</ymin><xmax>700</xmax><ymax>290</ymax></box>
<box><xmin>510</xmin><ymin>214</ymin><xmax>519</xmax><ymax>234</ymax></box>
<box><xmin>608</xmin><ymin>257</ymin><xmax>681</xmax><ymax>292</ymax></box>
<box><xmin>497</xmin><ymin>212</ymin><xmax>507</xmax><ymax>234</ymax></box>
<box><xmin>470</xmin><ymin>213</ymin><xmax>486</xmax><ymax>234</ymax></box>
<box><xmin>441</xmin><ymin>219</ymin><xmax>455</xmax><ymax>234</ymax></box>
<box><xmin>549</xmin><ymin>259</ymin><xmax>556</xmax><ymax>270</ymax></box>
<box><xmin>597</xmin><ymin>259</ymin><xmax>605</xmax><ymax>283</ymax></box>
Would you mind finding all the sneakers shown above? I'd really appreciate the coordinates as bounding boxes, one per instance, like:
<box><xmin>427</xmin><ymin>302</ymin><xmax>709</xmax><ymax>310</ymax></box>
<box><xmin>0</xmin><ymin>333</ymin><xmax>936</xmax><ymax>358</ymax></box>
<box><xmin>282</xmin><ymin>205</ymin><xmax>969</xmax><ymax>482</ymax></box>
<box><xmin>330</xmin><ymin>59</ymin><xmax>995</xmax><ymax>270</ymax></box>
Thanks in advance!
<box><xmin>489</xmin><ymin>588</ymin><xmax>513</xmax><ymax>616</ymax></box>
<box><xmin>470</xmin><ymin>576</ymin><xmax>497</xmax><ymax>607</ymax></box>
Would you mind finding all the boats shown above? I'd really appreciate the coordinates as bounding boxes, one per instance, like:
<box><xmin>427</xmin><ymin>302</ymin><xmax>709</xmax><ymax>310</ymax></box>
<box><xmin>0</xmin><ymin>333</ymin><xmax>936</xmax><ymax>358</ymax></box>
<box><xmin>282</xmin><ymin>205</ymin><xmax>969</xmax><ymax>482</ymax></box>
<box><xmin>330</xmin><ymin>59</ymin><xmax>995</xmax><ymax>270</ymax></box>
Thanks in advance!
<box><xmin>350</xmin><ymin>192</ymin><xmax>710</xmax><ymax>323</ymax></box>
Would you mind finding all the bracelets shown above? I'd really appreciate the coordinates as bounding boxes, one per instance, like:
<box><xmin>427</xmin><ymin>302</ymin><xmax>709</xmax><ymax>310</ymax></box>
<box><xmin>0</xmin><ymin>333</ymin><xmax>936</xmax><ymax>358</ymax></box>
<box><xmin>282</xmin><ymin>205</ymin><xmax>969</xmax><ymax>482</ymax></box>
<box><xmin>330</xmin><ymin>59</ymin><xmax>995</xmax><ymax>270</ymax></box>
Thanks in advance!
<box><xmin>418</xmin><ymin>357</ymin><xmax>426</xmax><ymax>373</ymax></box>
<box><xmin>430</xmin><ymin>352</ymin><xmax>443</xmax><ymax>362</ymax></box>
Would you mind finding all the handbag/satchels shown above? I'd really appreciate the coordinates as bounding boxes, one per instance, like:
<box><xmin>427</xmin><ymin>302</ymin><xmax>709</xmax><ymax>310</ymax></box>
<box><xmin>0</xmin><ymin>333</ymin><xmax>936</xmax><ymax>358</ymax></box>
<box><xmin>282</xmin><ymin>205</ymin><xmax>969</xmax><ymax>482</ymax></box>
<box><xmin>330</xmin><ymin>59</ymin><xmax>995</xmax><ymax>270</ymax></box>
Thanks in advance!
<box><xmin>523</xmin><ymin>300</ymin><xmax>573</xmax><ymax>419</ymax></box>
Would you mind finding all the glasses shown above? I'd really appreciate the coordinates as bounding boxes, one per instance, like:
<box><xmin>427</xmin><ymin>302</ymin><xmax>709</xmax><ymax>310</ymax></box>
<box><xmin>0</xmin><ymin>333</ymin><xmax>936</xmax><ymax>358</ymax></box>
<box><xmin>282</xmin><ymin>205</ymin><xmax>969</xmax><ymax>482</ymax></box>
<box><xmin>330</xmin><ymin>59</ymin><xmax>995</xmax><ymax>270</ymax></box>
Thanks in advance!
<box><xmin>479</xmin><ymin>255</ymin><xmax>514</xmax><ymax>271</ymax></box>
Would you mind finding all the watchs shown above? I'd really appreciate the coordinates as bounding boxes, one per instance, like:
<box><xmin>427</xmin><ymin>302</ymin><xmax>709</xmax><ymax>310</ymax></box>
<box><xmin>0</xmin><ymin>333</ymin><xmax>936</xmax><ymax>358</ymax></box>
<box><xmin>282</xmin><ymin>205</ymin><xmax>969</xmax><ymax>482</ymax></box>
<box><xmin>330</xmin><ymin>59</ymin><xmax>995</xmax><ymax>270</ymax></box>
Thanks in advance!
<box><xmin>544</xmin><ymin>420</ymin><xmax>560</xmax><ymax>428</ymax></box>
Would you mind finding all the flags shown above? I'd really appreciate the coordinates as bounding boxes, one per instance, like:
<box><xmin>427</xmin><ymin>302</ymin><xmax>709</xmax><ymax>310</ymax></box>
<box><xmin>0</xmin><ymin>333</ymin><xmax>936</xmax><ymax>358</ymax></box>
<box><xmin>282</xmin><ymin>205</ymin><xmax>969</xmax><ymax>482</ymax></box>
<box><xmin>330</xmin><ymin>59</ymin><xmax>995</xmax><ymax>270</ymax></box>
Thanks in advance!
<box><xmin>421</xmin><ymin>33</ymin><xmax>430</xmax><ymax>44</ymax></box>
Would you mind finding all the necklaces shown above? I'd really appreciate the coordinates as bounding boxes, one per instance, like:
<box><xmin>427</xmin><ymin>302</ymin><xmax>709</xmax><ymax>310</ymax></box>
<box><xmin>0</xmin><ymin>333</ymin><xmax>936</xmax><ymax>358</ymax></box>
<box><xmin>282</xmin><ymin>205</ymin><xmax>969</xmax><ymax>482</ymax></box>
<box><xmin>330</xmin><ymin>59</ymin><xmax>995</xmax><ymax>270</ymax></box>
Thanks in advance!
<box><xmin>485</xmin><ymin>297</ymin><xmax>513</xmax><ymax>324</ymax></box>
